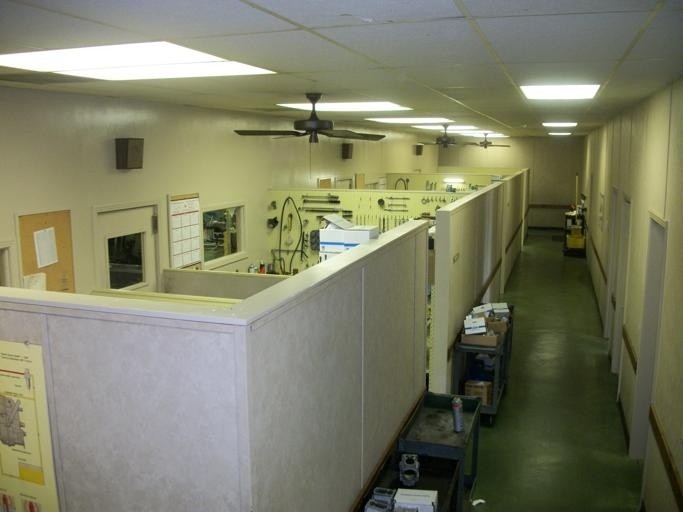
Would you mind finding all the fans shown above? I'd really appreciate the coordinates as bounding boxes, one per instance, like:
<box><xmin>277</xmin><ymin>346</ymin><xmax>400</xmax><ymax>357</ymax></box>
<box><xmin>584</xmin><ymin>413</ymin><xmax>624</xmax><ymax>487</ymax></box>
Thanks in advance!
<box><xmin>233</xmin><ymin>93</ymin><xmax>386</xmax><ymax>143</ymax></box>
<box><xmin>465</xmin><ymin>133</ymin><xmax>511</xmax><ymax>149</ymax></box>
<box><xmin>418</xmin><ymin>124</ymin><xmax>474</xmax><ymax>148</ymax></box>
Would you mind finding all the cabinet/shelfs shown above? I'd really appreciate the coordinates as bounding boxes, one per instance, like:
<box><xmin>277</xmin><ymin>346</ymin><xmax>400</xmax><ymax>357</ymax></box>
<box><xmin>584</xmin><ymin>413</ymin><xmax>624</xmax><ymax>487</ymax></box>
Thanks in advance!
<box><xmin>453</xmin><ymin>303</ymin><xmax>514</xmax><ymax>427</ymax></box>
<box><xmin>349</xmin><ymin>391</ymin><xmax>483</xmax><ymax>512</ymax></box>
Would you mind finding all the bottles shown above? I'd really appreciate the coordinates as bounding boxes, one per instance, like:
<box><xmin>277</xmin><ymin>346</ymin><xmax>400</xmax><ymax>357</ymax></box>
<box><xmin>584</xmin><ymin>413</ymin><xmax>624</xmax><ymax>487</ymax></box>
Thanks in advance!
<box><xmin>449</xmin><ymin>395</ymin><xmax>464</xmax><ymax>435</ymax></box>
<box><xmin>234</xmin><ymin>260</ymin><xmax>276</xmax><ymax>274</ymax></box>
